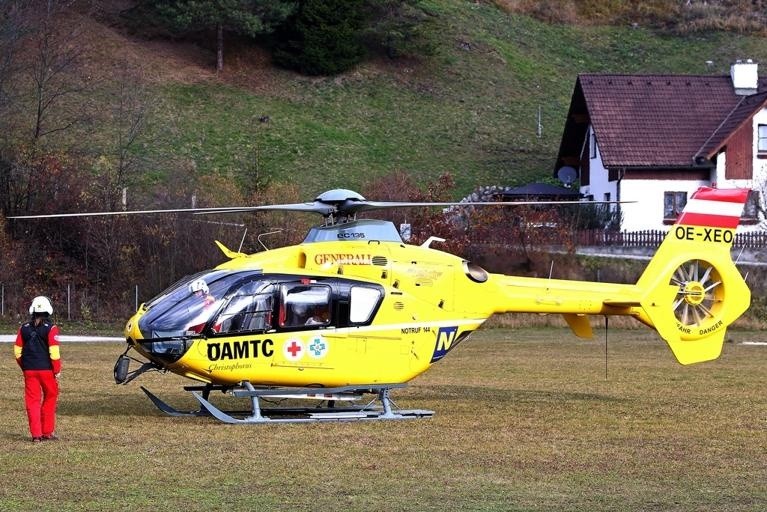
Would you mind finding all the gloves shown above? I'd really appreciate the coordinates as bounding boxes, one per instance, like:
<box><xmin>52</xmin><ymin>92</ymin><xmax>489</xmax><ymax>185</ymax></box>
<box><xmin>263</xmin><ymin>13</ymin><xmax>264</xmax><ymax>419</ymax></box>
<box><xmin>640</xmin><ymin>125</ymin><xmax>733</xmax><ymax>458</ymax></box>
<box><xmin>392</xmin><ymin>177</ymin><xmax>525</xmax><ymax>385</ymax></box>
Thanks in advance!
<box><xmin>55</xmin><ymin>372</ymin><xmax>61</xmax><ymax>378</ymax></box>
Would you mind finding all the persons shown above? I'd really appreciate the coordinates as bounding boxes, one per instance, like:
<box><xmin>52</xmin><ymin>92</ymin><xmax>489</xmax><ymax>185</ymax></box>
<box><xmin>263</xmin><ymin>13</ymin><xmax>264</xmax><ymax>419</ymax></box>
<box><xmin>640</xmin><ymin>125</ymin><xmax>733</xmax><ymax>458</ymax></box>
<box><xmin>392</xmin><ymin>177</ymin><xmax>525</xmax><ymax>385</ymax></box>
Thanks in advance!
<box><xmin>304</xmin><ymin>303</ymin><xmax>329</xmax><ymax>325</ymax></box>
<box><xmin>188</xmin><ymin>279</ymin><xmax>222</xmax><ymax>332</ymax></box>
<box><xmin>291</xmin><ymin>300</ymin><xmax>307</xmax><ymax>326</ymax></box>
<box><xmin>13</xmin><ymin>296</ymin><xmax>61</xmax><ymax>442</ymax></box>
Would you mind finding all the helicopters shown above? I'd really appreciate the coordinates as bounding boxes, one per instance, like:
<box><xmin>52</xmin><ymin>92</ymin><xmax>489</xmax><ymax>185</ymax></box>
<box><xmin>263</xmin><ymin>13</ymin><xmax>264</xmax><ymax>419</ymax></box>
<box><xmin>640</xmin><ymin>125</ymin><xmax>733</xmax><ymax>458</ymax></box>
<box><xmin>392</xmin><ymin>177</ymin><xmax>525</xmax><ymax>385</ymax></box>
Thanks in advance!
<box><xmin>6</xmin><ymin>180</ymin><xmax>751</xmax><ymax>424</ymax></box>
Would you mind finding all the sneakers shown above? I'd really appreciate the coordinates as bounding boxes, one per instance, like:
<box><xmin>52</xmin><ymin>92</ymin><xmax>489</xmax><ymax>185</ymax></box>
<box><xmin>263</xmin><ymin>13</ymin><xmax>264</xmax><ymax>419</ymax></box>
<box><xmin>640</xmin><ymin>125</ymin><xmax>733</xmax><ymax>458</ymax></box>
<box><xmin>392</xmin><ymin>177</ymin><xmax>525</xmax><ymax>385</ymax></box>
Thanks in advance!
<box><xmin>31</xmin><ymin>434</ymin><xmax>59</xmax><ymax>443</ymax></box>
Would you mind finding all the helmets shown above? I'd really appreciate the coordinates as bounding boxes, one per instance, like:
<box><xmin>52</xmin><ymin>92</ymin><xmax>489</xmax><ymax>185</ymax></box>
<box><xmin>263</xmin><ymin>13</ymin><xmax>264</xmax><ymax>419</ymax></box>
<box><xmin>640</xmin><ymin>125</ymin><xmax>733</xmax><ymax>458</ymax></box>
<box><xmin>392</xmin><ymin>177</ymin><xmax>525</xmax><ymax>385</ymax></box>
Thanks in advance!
<box><xmin>189</xmin><ymin>279</ymin><xmax>209</xmax><ymax>294</ymax></box>
<box><xmin>29</xmin><ymin>296</ymin><xmax>53</xmax><ymax>315</ymax></box>
